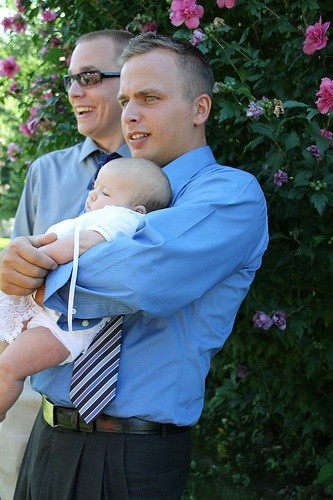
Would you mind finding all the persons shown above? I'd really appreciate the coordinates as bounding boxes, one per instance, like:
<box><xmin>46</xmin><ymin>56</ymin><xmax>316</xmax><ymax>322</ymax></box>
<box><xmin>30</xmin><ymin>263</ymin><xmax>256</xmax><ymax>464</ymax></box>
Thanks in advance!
<box><xmin>0</xmin><ymin>156</ymin><xmax>174</xmax><ymax>422</ymax></box>
<box><xmin>9</xmin><ymin>31</ymin><xmax>135</xmax><ymax>240</ymax></box>
<box><xmin>0</xmin><ymin>30</ymin><xmax>270</xmax><ymax>500</ymax></box>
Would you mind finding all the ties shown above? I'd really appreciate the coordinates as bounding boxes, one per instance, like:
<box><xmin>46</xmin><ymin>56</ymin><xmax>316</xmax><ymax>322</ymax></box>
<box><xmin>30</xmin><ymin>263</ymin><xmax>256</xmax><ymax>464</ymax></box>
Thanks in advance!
<box><xmin>78</xmin><ymin>153</ymin><xmax>122</xmax><ymax>216</ymax></box>
<box><xmin>69</xmin><ymin>315</ymin><xmax>125</xmax><ymax>424</ymax></box>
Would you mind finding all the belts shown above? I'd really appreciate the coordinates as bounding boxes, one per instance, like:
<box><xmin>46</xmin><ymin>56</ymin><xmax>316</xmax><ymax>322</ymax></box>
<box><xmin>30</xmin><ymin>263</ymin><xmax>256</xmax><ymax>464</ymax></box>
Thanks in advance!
<box><xmin>40</xmin><ymin>394</ymin><xmax>189</xmax><ymax>435</ymax></box>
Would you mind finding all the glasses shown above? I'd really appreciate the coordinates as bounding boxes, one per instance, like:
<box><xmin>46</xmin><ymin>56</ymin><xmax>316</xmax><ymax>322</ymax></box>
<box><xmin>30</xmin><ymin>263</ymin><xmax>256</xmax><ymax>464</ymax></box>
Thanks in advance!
<box><xmin>63</xmin><ymin>70</ymin><xmax>121</xmax><ymax>92</ymax></box>
